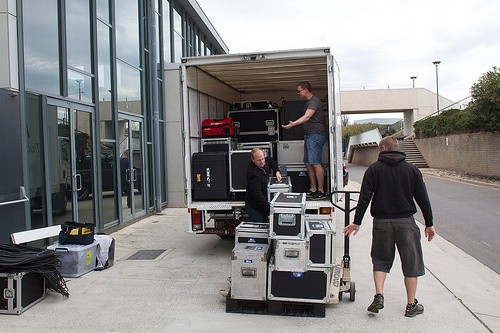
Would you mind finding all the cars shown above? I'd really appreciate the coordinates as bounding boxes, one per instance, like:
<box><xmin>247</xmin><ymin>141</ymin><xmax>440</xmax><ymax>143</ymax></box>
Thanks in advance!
<box><xmin>64</xmin><ymin>159</ymin><xmax>143</xmax><ymax>201</ymax></box>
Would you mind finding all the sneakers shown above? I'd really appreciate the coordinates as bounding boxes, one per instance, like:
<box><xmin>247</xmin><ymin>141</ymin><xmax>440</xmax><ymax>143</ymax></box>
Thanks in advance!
<box><xmin>310</xmin><ymin>191</ymin><xmax>326</xmax><ymax>200</ymax></box>
<box><xmin>405</xmin><ymin>298</ymin><xmax>424</xmax><ymax>317</ymax></box>
<box><xmin>366</xmin><ymin>293</ymin><xmax>384</xmax><ymax>313</ymax></box>
<box><xmin>306</xmin><ymin>188</ymin><xmax>317</xmax><ymax>197</ymax></box>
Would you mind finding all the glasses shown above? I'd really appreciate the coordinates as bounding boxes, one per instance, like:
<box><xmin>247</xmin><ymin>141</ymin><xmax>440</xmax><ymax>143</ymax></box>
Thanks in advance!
<box><xmin>297</xmin><ymin>89</ymin><xmax>304</xmax><ymax>93</ymax></box>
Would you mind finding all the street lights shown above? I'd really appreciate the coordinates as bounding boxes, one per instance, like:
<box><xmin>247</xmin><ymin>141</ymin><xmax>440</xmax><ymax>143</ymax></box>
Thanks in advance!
<box><xmin>432</xmin><ymin>61</ymin><xmax>442</xmax><ymax>115</ymax></box>
<box><xmin>76</xmin><ymin>79</ymin><xmax>83</xmax><ymax>99</ymax></box>
<box><xmin>410</xmin><ymin>76</ymin><xmax>417</xmax><ymax>88</ymax></box>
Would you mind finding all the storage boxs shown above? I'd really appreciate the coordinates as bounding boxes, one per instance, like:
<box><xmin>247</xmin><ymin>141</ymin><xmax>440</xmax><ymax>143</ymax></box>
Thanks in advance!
<box><xmin>201</xmin><ymin>137</ymin><xmax>238</xmax><ymax>152</ymax></box>
<box><xmin>0</xmin><ymin>270</ymin><xmax>45</xmax><ymax>314</ymax></box>
<box><xmin>47</xmin><ymin>244</ymin><xmax>97</xmax><ymax>277</ymax></box>
<box><xmin>231</xmin><ymin>192</ymin><xmax>336</xmax><ymax>304</ymax></box>
<box><xmin>227</xmin><ymin>100</ymin><xmax>280</xmax><ymax>199</ymax></box>
<box><xmin>276</xmin><ymin>140</ymin><xmax>330</xmax><ymax>196</ymax></box>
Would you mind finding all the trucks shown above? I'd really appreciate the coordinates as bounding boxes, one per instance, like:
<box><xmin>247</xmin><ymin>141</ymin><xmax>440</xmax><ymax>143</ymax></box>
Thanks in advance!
<box><xmin>163</xmin><ymin>49</ymin><xmax>346</xmax><ymax>242</ymax></box>
<box><xmin>0</xmin><ymin>84</ymin><xmax>71</xmax><ymax>217</ymax></box>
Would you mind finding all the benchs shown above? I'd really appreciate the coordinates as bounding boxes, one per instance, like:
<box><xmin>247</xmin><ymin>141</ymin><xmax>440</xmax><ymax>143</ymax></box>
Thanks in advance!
<box><xmin>11</xmin><ymin>224</ymin><xmax>62</xmax><ymax>250</ymax></box>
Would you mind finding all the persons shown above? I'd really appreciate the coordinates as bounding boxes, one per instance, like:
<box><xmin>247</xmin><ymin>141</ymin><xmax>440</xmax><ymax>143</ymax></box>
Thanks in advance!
<box><xmin>342</xmin><ymin>136</ymin><xmax>434</xmax><ymax>317</ymax></box>
<box><xmin>282</xmin><ymin>81</ymin><xmax>327</xmax><ymax>199</ymax></box>
<box><xmin>120</xmin><ymin>150</ymin><xmax>129</xmax><ymax>186</ymax></box>
<box><xmin>245</xmin><ymin>148</ymin><xmax>282</xmax><ymax>223</ymax></box>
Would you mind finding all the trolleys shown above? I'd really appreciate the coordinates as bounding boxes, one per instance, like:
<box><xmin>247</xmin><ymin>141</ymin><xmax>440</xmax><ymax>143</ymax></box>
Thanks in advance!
<box><xmin>222</xmin><ymin>190</ymin><xmax>361</xmax><ymax>309</ymax></box>
